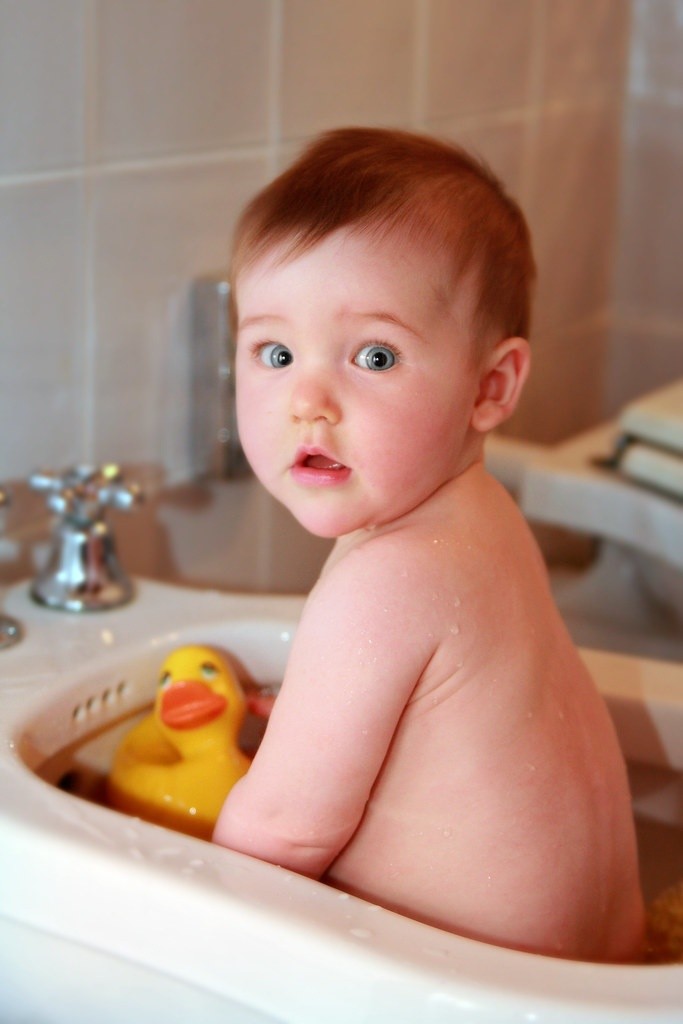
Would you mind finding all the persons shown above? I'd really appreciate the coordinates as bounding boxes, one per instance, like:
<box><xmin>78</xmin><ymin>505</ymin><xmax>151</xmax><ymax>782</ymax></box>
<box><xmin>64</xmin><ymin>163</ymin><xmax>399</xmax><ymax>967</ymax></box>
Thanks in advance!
<box><xmin>211</xmin><ymin>126</ymin><xmax>644</xmax><ymax>958</ymax></box>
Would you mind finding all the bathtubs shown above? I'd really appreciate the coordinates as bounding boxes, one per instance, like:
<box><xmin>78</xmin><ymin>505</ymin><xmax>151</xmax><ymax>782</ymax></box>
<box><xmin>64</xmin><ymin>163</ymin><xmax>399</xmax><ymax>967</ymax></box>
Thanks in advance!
<box><xmin>0</xmin><ymin>575</ymin><xmax>683</xmax><ymax>1024</ymax></box>
